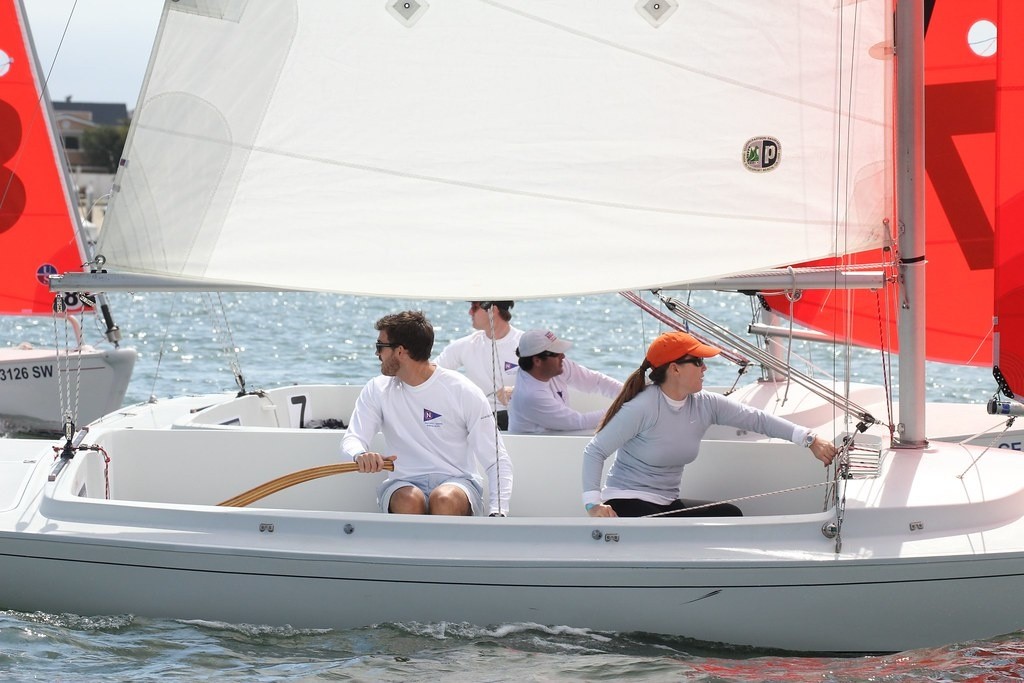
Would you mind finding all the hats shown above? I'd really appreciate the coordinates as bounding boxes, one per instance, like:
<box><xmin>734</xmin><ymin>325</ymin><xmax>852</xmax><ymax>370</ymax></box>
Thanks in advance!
<box><xmin>646</xmin><ymin>332</ymin><xmax>722</xmax><ymax>370</ymax></box>
<box><xmin>519</xmin><ymin>329</ymin><xmax>573</xmax><ymax>358</ymax></box>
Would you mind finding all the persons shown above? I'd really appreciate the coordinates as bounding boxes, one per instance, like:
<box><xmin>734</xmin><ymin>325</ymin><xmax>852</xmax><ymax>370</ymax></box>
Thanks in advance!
<box><xmin>508</xmin><ymin>328</ymin><xmax>624</xmax><ymax>433</ymax></box>
<box><xmin>341</xmin><ymin>311</ymin><xmax>513</xmax><ymax>517</ymax></box>
<box><xmin>432</xmin><ymin>301</ymin><xmax>524</xmax><ymax>431</ymax></box>
<box><xmin>582</xmin><ymin>331</ymin><xmax>838</xmax><ymax>517</ymax></box>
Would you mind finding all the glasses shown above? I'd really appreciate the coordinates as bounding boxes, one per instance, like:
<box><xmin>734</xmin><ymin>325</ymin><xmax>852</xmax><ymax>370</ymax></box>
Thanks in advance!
<box><xmin>375</xmin><ymin>343</ymin><xmax>407</xmax><ymax>353</ymax></box>
<box><xmin>666</xmin><ymin>356</ymin><xmax>702</xmax><ymax>367</ymax></box>
<box><xmin>536</xmin><ymin>352</ymin><xmax>559</xmax><ymax>357</ymax></box>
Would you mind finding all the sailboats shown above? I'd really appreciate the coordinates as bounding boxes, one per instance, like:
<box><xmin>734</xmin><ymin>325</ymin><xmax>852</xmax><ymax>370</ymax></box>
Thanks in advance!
<box><xmin>0</xmin><ymin>1</ymin><xmax>1024</xmax><ymax>656</ymax></box>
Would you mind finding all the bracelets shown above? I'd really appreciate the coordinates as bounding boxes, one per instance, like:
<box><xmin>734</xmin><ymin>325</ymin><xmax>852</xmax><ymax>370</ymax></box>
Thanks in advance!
<box><xmin>586</xmin><ymin>504</ymin><xmax>593</xmax><ymax>510</ymax></box>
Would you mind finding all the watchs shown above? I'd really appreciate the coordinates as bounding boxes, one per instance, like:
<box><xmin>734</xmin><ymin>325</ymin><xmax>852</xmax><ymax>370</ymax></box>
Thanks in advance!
<box><xmin>804</xmin><ymin>433</ymin><xmax>817</xmax><ymax>447</ymax></box>
